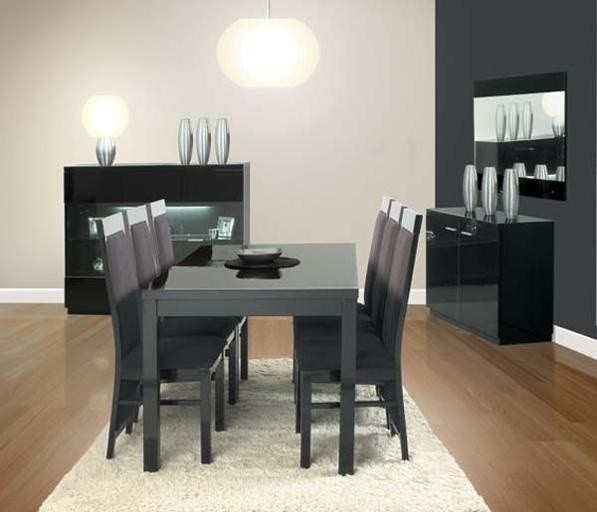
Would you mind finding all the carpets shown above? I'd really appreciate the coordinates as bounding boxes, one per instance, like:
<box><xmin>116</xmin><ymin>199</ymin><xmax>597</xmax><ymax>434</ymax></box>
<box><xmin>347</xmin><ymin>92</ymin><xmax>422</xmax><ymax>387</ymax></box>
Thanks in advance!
<box><xmin>38</xmin><ymin>358</ymin><xmax>492</xmax><ymax>511</ymax></box>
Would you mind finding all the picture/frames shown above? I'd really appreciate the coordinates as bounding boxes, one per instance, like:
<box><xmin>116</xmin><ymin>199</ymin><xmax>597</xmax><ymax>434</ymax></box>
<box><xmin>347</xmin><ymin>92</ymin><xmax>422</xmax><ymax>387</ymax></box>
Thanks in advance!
<box><xmin>217</xmin><ymin>216</ymin><xmax>234</xmax><ymax>238</ymax></box>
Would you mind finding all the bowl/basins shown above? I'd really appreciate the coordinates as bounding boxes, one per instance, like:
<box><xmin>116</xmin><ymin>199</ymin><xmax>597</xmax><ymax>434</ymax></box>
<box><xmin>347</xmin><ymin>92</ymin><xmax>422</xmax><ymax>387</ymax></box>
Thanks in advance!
<box><xmin>236</xmin><ymin>249</ymin><xmax>281</xmax><ymax>264</ymax></box>
<box><xmin>236</xmin><ymin>270</ymin><xmax>281</xmax><ymax>279</ymax></box>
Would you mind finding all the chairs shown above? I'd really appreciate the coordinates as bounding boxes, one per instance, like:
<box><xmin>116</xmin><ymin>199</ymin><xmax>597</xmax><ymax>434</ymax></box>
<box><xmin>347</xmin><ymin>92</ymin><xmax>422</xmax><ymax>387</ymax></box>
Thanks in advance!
<box><xmin>147</xmin><ymin>199</ymin><xmax>249</xmax><ymax>380</ymax></box>
<box><xmin>123</xmin><ymin>205</ymin><xmax>238</xmax><ymax>404</ymax></box>
<box><xmin>293</xmin><ymin>196</ymin><xmax>423</xmax><ymax>470</ymax></box>
<box><xmin>97</xmin><ymin>213</ymin><xmax>225</xmax><ymax>463</ymax></box>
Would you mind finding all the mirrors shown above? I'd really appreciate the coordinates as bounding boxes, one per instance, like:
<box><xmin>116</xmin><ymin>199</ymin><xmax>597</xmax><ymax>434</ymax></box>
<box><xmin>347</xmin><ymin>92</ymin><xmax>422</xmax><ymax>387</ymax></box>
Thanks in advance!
<box><xmin>473</xmin><ymin>70</ymin><xmax>568</xmax><ymax>201</ymax></box>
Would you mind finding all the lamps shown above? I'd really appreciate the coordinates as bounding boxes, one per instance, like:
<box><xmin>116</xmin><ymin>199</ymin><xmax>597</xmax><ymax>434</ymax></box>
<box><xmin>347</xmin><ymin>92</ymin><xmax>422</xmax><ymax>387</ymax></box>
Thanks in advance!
<box><xmin>215</xmin><ymin>1</ymin><xmax>320</xmax><ymax>90</ymax></box>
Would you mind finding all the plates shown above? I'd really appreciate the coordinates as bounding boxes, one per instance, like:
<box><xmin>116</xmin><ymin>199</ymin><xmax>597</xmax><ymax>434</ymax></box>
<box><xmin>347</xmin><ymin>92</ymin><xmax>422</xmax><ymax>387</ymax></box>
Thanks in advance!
<box><xmin>225</xmin><ymin>258</ymin><xmax>300</xmax><ymax>269</ymax></box>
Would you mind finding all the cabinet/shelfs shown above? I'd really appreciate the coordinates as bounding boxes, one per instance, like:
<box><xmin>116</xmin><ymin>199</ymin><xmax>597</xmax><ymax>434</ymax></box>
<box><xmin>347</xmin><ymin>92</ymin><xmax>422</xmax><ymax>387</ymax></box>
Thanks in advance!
<box><xmin>64</xmin><ymin>162</ymin><xmax>250</xmax><ymax>316</ymax></box>
<box><xmin>426</xmin><ymin>204</ymin><xmax>555</xmax><ymax>346</ymax></box>
